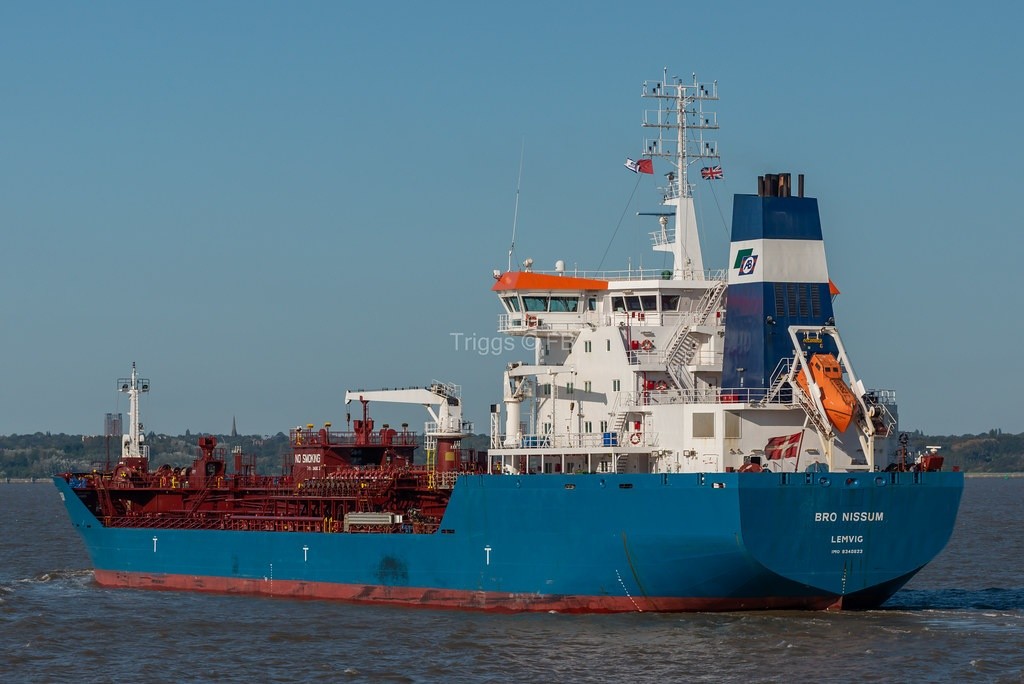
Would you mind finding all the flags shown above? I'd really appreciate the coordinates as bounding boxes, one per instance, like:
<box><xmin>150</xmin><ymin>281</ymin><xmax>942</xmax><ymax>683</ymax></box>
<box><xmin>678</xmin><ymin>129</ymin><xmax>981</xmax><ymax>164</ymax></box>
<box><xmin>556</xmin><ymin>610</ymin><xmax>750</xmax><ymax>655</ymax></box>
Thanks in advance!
<box><xmin>701</xmin><ymin>165</ymin><xmax>725</xmax><ymax>180</ymax></box>
<box><xmin>624</xmin><ymin>158</ymin><xmax>654</xmax><ymax>174</ymax></box>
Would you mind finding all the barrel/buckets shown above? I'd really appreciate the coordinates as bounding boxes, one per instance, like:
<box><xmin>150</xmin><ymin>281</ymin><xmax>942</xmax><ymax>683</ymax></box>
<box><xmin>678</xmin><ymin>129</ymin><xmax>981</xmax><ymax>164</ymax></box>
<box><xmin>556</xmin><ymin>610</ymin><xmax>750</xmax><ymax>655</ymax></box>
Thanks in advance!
<box><xmin>604</xmin><ymin>433</ymin><xmax>617</xmax><ymax>447</ymax></box>
<box><xmin>521</xmin><ymin>436</ymin><xmax>544</xmax><ymax>448</ymax></box>
<box><xmin>720</xmin><ymin>393</ymin><xmax>738</xmax><ymax>403</ymax></box>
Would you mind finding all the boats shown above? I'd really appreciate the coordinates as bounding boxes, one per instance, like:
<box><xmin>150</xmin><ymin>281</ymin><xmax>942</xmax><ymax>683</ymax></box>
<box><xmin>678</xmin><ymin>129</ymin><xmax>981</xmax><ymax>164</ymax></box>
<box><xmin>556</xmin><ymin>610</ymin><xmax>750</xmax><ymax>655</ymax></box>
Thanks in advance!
<box><xmin>796</xmin><ymin>352</ymin><xmax>859</xmax><ymax>434</ymax></box>
<box><xmin>52</xmin><ymin>64</ymin><xmax>966</xmax><ymax>613</ymax></box>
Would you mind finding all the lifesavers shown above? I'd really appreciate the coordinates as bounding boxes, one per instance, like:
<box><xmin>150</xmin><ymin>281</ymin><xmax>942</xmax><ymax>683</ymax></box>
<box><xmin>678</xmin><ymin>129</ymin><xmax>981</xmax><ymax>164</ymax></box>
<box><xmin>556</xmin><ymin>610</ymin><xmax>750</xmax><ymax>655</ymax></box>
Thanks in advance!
<box><xmin>899</xmin><ymin>433</ymin><xmax>908</xmax><ymax>444</ymax></box>
<box><xmin>642</xmin><ymin>340</ymin><xmax>651</xmax><ymax>350</ymax></box>
<box><xmin>630</xmin><ymin>434</ymin><xmax>640</xmax><ymax>444</ymax></box>
<box><xmin>656</xmin><ymin>380</ymin><xmax>667</xmax><ymax>390</ymax></box>
